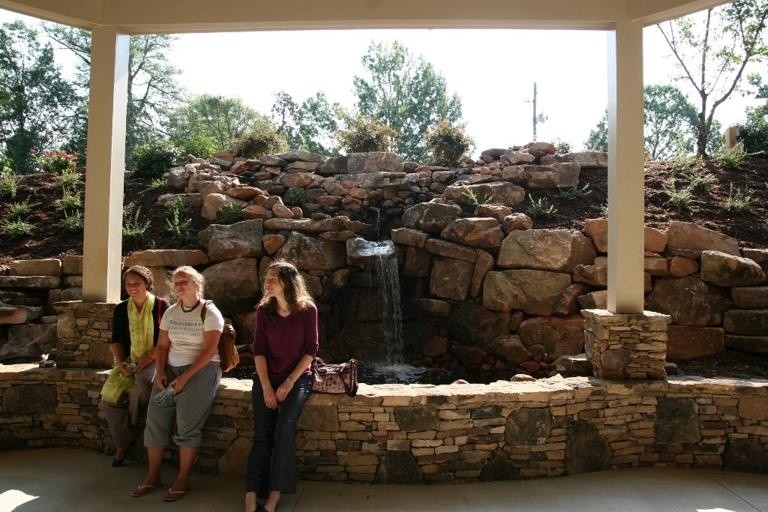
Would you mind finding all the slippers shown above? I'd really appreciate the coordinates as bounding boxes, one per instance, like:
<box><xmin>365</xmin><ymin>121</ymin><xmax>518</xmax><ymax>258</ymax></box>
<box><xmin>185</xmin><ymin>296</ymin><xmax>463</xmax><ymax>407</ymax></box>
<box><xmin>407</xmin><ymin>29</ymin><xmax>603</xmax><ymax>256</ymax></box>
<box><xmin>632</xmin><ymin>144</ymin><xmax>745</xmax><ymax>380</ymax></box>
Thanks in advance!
<box><xmin>131</xmin><ymin>483</ymin><xmax>156</xmax><ymax>497</ymax></box>
<box><xmin>163</xmin><ymin>487</ymin><xmax>190</xmax><ymax>501</ymax></box>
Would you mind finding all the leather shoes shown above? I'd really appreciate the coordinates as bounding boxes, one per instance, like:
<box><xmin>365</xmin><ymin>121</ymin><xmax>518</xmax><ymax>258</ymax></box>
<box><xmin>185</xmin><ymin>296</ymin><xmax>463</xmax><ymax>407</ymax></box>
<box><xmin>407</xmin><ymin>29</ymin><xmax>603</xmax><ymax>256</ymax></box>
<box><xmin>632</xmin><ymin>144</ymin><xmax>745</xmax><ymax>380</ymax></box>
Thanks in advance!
<box><xmin>111</xmin><ymin>441</ymin><xmax>135</xmax><ymax>467</ymax></box>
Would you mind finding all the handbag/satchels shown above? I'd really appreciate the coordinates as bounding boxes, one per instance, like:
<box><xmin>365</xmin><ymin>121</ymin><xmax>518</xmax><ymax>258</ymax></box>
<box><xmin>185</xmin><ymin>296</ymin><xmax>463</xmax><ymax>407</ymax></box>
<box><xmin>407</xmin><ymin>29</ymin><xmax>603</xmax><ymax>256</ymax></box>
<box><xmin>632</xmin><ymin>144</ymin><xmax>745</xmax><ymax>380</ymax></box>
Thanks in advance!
<box><xmin>312</xmin><ymin>357</ymin><xmax>358</xmax><ymax>398</ymax></box>
<box><xmin>219</xmin><ymin>323</ymin><xmax>240</xmax><ymax>373</ymax></box>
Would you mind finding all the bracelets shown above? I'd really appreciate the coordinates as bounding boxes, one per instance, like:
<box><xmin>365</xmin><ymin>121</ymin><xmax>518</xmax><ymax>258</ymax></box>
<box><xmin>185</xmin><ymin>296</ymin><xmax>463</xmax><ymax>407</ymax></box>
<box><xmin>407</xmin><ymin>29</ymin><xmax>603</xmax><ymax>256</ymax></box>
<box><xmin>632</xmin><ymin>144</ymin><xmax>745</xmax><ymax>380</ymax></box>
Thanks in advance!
<box><xmin>287</xmin><ymin>377</ymin><xmax>294</xmax><ymax>384</ymax></box>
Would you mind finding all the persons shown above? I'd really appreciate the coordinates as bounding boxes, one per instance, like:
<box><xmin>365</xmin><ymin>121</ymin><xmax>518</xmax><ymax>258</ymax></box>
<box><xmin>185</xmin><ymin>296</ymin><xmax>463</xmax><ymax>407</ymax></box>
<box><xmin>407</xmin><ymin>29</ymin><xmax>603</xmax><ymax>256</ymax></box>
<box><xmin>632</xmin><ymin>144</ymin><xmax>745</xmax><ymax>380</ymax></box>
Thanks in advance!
<box><xmin>132</xmin><ymin>265</ymin><xmax>225</xmax><ymax>502</ymax></box>
<box><xmin>100</xmin><ymin>266</ymin><xmax>171</xmax><ymax>467</ymax></box>
<box><xmin>243</xmin><ymin>261</ymin><xmax>319</xmax><ymax>512</ymax></box>
